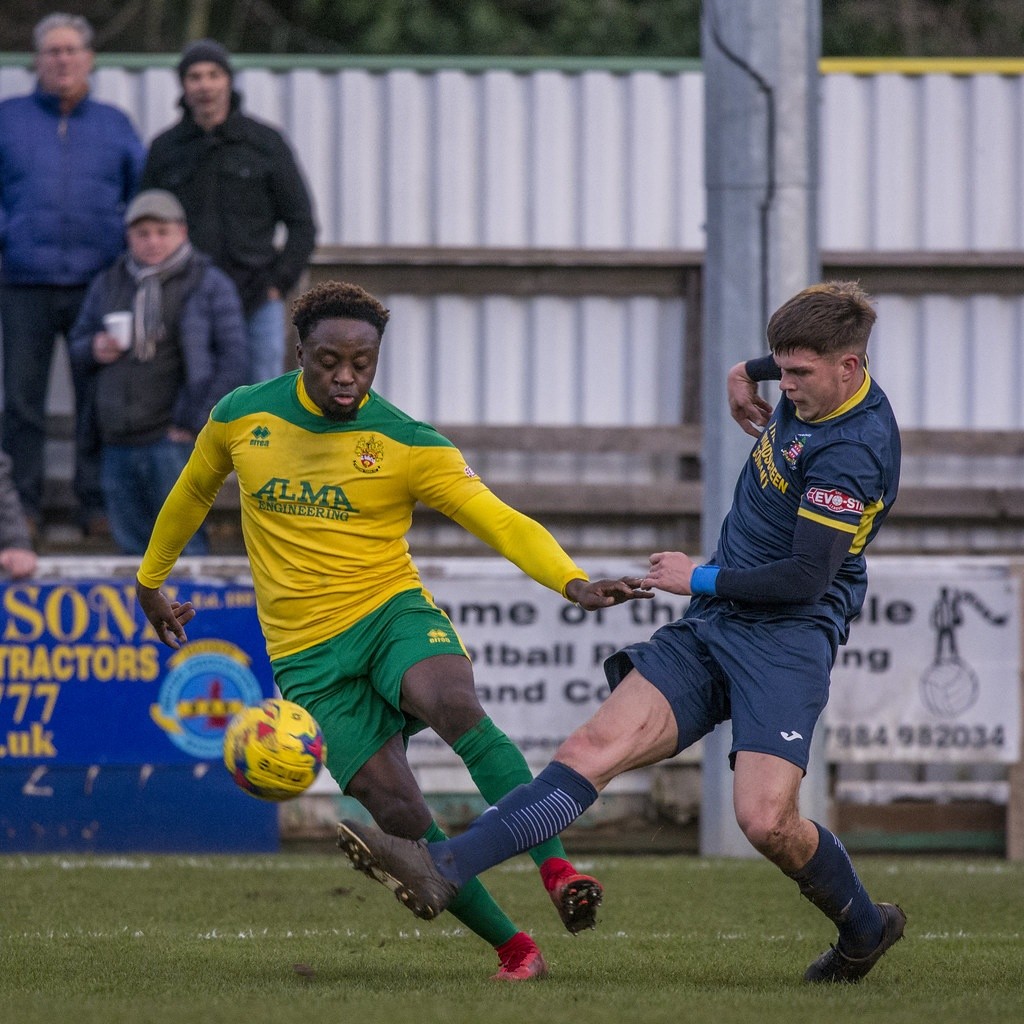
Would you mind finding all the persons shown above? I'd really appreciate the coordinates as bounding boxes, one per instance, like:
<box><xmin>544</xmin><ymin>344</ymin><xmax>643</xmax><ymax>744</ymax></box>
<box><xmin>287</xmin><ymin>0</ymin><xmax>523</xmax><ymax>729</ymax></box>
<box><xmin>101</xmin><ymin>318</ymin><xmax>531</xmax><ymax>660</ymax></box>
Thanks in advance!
<box><xmin>140</xmin><ymin>41</ymin><xmax>316</xmax><ymax>384</ymax></box>
<box><xmin>0</xmin><ymin>448</ymin><xmax>38</xmax><ymax>579</ymax></box>
<box><xmin>136</xmin><ymin>283</ymin><xmax>656</xmax><ymax>982</ymax></box>
<box><xmin>71</xmin><ymin>188</ymin><xmax>250</xmax><ymax>555</ymax></box>
<box><xmin>336</xmin><ymin>281</ymin><xmax>909</xmax><ymax>982</ymax></box>
<box><xmin>0</xmin><ymin>13</ymin><xmax>149</xmax><ymax>548</ymax></box>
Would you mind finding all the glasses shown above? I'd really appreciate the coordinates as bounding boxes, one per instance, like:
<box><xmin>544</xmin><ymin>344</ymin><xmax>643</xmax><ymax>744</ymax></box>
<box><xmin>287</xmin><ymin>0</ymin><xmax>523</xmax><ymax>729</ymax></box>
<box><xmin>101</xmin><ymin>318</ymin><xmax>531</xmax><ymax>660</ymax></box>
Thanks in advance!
<box><xmin>39</xmin><ymin>44</ymin><xmax>88</xmax><ymax>56</ymax></box>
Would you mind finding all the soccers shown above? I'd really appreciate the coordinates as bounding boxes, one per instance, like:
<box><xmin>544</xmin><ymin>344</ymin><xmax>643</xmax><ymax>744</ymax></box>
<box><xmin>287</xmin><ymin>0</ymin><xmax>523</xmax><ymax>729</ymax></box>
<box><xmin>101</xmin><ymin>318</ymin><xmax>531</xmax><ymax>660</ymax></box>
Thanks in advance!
<box><xmin>222</xmin><ymin>697</ymin><xmax>329</xmax><ymax>806</ymax></box>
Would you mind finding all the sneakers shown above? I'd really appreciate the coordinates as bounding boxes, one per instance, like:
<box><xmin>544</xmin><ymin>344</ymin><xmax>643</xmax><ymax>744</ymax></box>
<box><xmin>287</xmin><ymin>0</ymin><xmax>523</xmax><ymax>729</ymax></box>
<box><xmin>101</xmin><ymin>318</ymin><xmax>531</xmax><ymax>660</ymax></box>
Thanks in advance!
<box><xmin>336</xmin><ymin>819</ymin><xmax>459</xmax><ymax>922</ymax></box>
<box><xmin>539</xmin><ymin>856</ymin><xmax>603</xmax><ymax>936</ymax></box>
<box><xmin>803</xmin><ymin>903</ymin><xmax>906</xmax><ymax>988</ymax></box>
<box><xmin>490</xmin><ymin>932</ymin><xmax>549</xmax><ymax>979</ymax></box>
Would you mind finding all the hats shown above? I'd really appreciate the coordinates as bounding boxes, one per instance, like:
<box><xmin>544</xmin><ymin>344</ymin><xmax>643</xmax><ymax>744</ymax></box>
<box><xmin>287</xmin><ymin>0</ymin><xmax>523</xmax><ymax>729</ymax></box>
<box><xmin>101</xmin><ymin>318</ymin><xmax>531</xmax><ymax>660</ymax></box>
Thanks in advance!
<box><xmin>177</xmin><ymin>39</ymin><xmax>233</xmax><ymax>80</ymax></box>
<box><xmin>124</xmin><ymin>189</ymin><xmax>185</xmax><ymax>228</ymax></box>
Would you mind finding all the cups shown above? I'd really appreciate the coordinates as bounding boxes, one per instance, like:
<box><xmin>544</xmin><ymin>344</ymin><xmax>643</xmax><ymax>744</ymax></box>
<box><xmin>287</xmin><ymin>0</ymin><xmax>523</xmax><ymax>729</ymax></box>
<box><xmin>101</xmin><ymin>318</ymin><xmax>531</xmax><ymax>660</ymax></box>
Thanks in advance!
<box><xmin>102</xmin><ymin>310</ymin><xmax>133</xmax><ymax>350</ymax></box>
